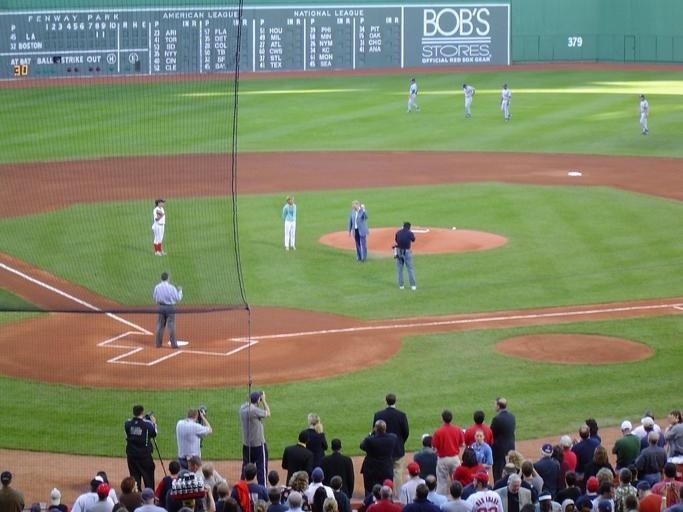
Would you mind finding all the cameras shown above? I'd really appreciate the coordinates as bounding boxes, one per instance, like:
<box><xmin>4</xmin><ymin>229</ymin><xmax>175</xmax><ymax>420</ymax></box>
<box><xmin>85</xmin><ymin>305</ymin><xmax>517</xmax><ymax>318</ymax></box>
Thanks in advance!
<box><xmin>144</xmin><ymin>411</ymin><xmax>153</xmax><ymax>420</ymax></box>
<box><xmin>258</xmin><ymin>391</ymin><xmax>263</xmax><ymax>401</ymax></box>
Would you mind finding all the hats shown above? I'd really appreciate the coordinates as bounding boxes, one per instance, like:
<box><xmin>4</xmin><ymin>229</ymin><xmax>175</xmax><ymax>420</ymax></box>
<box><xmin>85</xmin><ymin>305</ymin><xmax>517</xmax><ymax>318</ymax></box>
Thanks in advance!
<box><xmin>540</xmin><ymin>418</ymin><xmax>599</xmax><ymax>457</ymax></box>
<box><xmin>382</xmin><ymin>462</ymin><xmax>488</xmax><ymax>487</ymax></box>
<box><xmin>620</xmin><ymin>416</ymin><xmax>660</xmax><ymax>445</ymax></box>
<box><xmin>1</xmin><ymin>472</ymin><xmax>12</xmax><ymax>481</ymax></box>
<box><xmin>250</xmin><ymin>391</ymin><xmax>263</xmax><ymax>399</ymax></box>
<box><xmin>312</xmin><ymin>467</ymin><xmax>324</xmax><ymax>481</ymax></box>
<box><xmin>91</xmin><ymin>475</ymin><xmax>110</xmax><ymax>495</ymax></box>
<box><xmin>50</xmin><ymin>488</ymin><xmax>61</xmax><ymax>506</ymax></box>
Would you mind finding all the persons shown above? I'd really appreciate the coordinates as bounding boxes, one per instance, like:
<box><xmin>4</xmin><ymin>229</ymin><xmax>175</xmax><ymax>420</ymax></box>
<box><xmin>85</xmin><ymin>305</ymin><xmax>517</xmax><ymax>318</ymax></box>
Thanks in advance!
<box><xmin>48</xmin><ymin>488</ymin><xmax>68</xmax><ymax>512</ymax></box>
<box><xmin>406</xmin><ymin>78</ymin><xmax>420</xmax><ymax>112</ymax></box>
<box><xmin>348</xmin><ymin>200</ymin><xmax>368</xmax><ymax>262</ymax></box>
<box><xmin>240</xmin><ymin>393</ymin><xmax>271</xmax><ymax>487</ymax></box>
<box><xmin>373</xmin><ymin>394</ymin><xmax>409</xmax><ymax>499</ymax></box>
<box><xmin>31</xmin><ymin>503</ymin><xmax>41</xmax><ymax>512</ymax></box>
<box><xmin>462</xmin><ymin>84</ymin><xmax>475</xmax><ymax>118</ymax></box>
<box><xmin>320</xmin><ymin>438</ymin><xmax>355</xmax><ymax>500</ymax></box>
<box><xmin>306</xmin><ymin>413</ymin><xmax>327</xmax><ymax>468</ymax></box>
<box><xmin>494</xmin><ymin>410</ymin><xmax>683</xmax><ymax>512</ymax></box>
<box><xmin>415</xmin><ymin>437</ymin><xmax>437</xmax><ymax>479</ymax></box>
<box><xmin>176</xmin><ymin>410</ymin><xmax>212</xmax><ymax>470</ymax></box>
<box><xmin>282</xmin><ymin>196</ymin><xmax>296</xmax><ymax>251</ymax></box>
<box><xmin>465</xmin><ymin>412</ymin><xmax>494</xmax><ymax>472</ymax></box>
<box><xmin>72</xmin><ymin>471</ymin><xmax>127</xmax><ymax>512</ymax></box>
<box><xmin>360</xmin><ymin>448</ymin><xmax>503</xmax><ymax>512</ymax></box>
<box><xmin>490</xmin><ymin>397</ymin><xmax>516</xmax><ymax>483</ymax></box>
<box><xmin>124</xmin><ymin>405</ymin><xmax>158</xmax><ymax>491</ymax></box>
<box><xmin>360</xmin><ymin>420</ymin><xmax>398</xmax><ymax>497</ymax></box>
<box><xmin>121</xmin><ymin>477</ymin><xmax>167</xmax><ymax>512</ymax></box>
<box><xmin>501</xmin><ymin>83</ymin><xmax>512</xmax><ymax>122</ymax></box>
<box><xmin>0</xmin><ymin>471</ymin><xmax>24</xmax><ymax>512</ymax></box>
<box><xmin>151</xmin><ymin>199</ymin><xmax>167</xmax><ymax>256</ymax></box>
<box><xmin>156</xmin><ymin>455</ymin><xmax>353</xmax><ymax>512</ymax></box>
<box><xmin>395</xmin><ymin>222</ymin><xmax>416</xmax><ymax>291</ymax></box>
<box><xmin>639</xmin><ymin>94</ymin><xmax>649</xmax><ymax>135</ymax></box>
<box><xmin>431</xmin><ymin>411</ymin><xmax>464</xmax><ymax>494</ymax></box>
<box><xmin>153</xmin><ymin>272</ymin><xmax>183</xmax><ymax>348</ymax></box>
<box><xmin>283</xmin><ymin>433</ymin><xmax>314</xmax><ymax>486</ymax></box>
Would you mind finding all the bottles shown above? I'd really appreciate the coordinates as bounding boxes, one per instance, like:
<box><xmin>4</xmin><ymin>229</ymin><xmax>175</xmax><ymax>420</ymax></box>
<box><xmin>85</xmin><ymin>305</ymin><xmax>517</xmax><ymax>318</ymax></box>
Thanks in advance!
<box><xmin>170</xmin><ymin>471</ymin><xmax>203</xmax><ymax>495</ymax></box>
<box><xmin>372</xmin><ymin>495</ymin><xmax>377</xmax><ymax>505</ymax></box>
